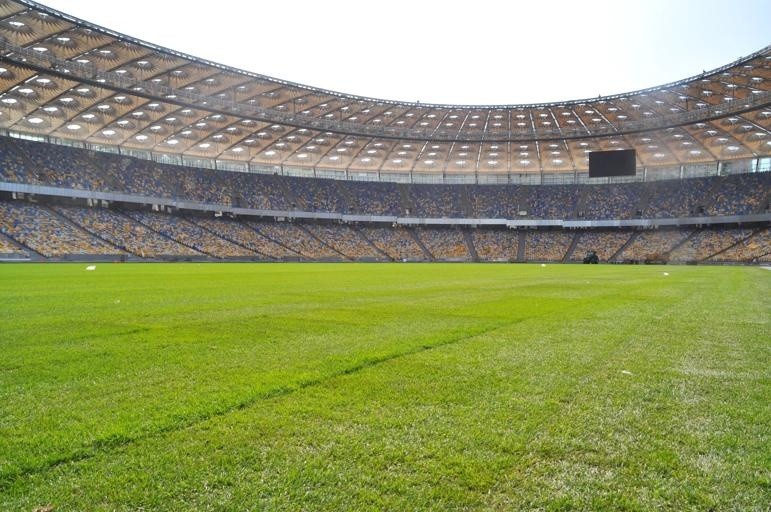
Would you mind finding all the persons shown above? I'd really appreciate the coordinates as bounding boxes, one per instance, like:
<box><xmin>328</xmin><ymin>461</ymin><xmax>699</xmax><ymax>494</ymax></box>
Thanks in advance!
<box><xmin>1</xmin><ymin>137</ymin><xmax>771</xmax><ymax>261</ymax></box>
